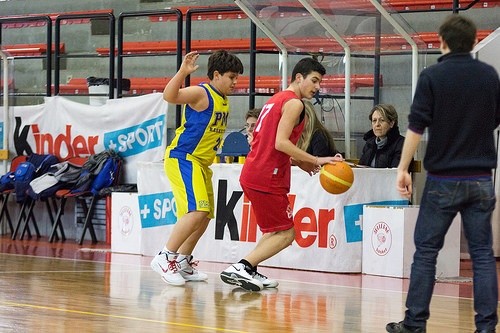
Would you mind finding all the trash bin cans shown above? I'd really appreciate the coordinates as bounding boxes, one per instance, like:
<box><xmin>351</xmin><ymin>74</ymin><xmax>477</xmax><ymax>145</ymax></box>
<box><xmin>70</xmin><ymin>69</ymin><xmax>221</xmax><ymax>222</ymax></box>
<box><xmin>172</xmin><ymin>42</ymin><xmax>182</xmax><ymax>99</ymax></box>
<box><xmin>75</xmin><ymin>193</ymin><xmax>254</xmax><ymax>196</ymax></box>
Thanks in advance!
<box><xmin>86</xmin><ymin>76</ymin><xmax>130</xmax><ymax>105</ymax></box>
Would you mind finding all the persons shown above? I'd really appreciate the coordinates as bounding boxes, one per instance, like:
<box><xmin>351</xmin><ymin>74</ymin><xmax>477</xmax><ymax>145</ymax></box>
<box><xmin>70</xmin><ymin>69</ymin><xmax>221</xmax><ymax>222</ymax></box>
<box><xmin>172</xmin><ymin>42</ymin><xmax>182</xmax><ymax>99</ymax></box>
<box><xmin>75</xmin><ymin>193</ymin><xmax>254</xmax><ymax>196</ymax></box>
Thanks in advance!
<box><xmin>220</xmin><ymin>58</ymin><xmax>343</xmax><ymax>291</ymax></box>
<box><xmin>151</xmin><ymin>51</ymin><xmax>244</xmax><ymax>285</ymax></box>
<box><xmin>296</xmin><ymin>100</ymin><xmax>336</xmax><ymax>156</ymax></box>
<box><xmin>245</xmin><ymin>110</ymin><xmax>262</xmax><ymax>146</ymax></box>
<box><xmin>358</xmin><ymin>104</ymin><xmax>414</xmax><ymax>172</ymax></box>
<box><xmin>386</xmin><ymin>16</ymin><xmax>500</xmax><ymax>333</ymax></box>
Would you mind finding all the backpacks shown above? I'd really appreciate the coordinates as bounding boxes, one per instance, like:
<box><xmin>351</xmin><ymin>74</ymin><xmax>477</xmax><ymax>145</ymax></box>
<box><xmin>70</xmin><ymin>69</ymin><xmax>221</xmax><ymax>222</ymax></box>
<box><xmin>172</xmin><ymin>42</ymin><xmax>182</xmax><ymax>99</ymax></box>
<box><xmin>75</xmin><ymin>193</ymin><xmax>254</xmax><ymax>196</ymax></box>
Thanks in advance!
<box><xmin>0</xmin><ymin>150</ymin><xmax>124</xmax><ymax>197</ymax></box>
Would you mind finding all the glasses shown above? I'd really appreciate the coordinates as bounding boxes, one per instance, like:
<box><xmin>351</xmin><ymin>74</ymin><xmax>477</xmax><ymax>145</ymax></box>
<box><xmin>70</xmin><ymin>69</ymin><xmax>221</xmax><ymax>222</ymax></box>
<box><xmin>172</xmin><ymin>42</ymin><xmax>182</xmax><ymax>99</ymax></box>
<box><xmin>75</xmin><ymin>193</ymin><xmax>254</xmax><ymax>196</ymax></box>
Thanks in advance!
<box><xmin>244</xmin><ymin>125</ymin><xmax>257</xmax><ymax>131</ymax></box>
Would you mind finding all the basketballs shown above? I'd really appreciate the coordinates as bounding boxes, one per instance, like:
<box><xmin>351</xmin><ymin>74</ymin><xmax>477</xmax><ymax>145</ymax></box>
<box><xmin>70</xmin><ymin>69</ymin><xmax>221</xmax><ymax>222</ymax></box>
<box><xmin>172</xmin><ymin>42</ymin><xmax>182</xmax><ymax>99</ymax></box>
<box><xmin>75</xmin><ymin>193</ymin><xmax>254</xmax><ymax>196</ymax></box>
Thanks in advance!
<box><xmin>319</xmin><ymin>161</ymin><xmax>354</xmax><ymax>195</ymax></box>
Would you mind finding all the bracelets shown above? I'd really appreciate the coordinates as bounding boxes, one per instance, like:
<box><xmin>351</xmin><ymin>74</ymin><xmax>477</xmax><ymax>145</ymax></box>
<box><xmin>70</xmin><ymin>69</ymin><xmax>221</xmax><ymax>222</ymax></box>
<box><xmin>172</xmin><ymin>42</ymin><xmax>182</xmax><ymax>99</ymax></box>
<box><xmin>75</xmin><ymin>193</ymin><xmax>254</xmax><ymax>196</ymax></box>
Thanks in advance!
<box><xmin>315</xmin><ymin>156</ymin><xmax>318</xmax><ymax>165</ymax></box>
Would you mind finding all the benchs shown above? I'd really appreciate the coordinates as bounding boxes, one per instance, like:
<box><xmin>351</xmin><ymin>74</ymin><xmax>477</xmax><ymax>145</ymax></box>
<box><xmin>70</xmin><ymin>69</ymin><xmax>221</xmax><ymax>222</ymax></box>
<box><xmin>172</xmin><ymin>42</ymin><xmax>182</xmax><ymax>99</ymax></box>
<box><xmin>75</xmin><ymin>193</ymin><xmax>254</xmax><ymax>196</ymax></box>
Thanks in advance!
<box><xmin>0</xmin><ymin>0</ymin><xmax>500</xmax><ymax>156</ymax></box>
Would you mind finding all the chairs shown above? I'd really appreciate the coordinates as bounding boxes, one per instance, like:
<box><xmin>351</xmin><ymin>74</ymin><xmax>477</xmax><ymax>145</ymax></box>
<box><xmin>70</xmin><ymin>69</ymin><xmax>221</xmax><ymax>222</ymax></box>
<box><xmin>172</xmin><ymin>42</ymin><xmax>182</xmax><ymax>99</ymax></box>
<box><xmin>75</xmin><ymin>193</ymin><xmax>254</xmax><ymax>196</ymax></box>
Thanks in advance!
<box><xmin>0</xmin><ymin>155</ymin><xmax>120</xmax><ymax>245</ymax></box>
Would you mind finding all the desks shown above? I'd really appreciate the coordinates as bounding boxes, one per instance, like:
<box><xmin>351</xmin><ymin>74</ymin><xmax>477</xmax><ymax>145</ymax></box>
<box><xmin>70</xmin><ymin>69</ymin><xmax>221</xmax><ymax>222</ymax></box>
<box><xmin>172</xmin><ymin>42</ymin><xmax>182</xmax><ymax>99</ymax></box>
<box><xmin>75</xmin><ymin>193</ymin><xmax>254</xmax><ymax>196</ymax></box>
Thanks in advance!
<box><xmin>360</xmin><ymin>202</ymin><xmax>461</xmax><ymax>278</ymax></box>
<box><xmin>138</xmin><ymin>162</ymin><xmax>404</xmax><ymax>274</ymax></box>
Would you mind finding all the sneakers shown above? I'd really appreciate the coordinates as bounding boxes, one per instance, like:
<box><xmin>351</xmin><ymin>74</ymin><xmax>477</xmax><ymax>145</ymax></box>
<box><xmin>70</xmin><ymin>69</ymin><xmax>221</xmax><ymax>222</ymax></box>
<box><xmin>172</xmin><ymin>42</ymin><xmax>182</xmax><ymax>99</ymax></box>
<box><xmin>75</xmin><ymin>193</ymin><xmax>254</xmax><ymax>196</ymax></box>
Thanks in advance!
<box><xmin>249</xmin><ymin>269</ymin><xmax>279</xmax><ymax>288</ymax></box>
<box><xmin>387</xmin><ymin>320</ymin><xmax>426</xmax><ymax>333</ymax></box>
<box><xmin>221</xmin><ymin>264</ymin><xmax>263</xmax><ymax>292</ymax></box>
<box><xmin>175</xmin><ymin>256</ymin><xmax>208</xmax><ymax>281</ymax></box>
<box><xmin>150</xmin><ymin>250</ymin><xmax>185</xmax><ymax>285</ymax></box>
